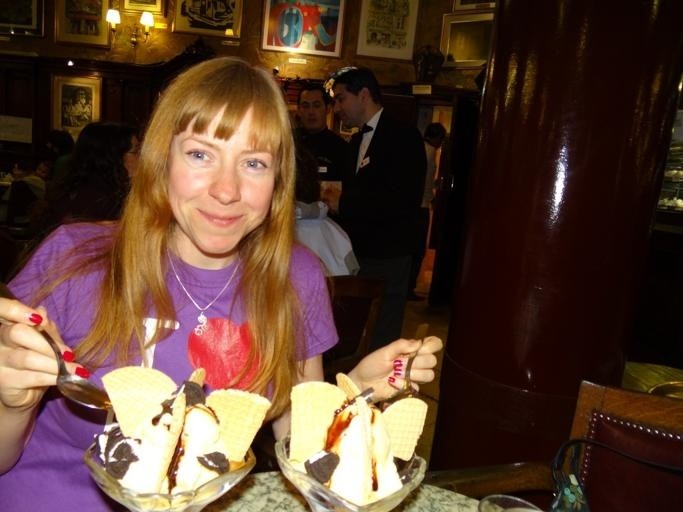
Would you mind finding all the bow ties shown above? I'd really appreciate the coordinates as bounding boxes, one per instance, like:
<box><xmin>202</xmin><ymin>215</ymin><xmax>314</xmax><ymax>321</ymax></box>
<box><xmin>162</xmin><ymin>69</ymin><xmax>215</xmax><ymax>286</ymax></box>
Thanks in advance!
<box><xmin>351</xmin><ymin>124</ymin><xmax>373</xmax><ymax>143</ymax></box>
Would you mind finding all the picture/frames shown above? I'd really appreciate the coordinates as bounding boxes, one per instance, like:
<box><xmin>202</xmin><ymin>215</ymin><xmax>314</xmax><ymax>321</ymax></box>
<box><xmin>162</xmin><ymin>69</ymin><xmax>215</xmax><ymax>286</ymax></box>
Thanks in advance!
<box><xmin>124</xmin><ymin>0</ymin><xmax>165</xmax><ymax>16</ymax></box>
<box><xmin>438</xmin><ymin>13</ymin><xmax>494</xmax><ymax>70</ymax></box>
<box><xmin>51</xmin><ymin>72</ymin><xmax>103</xmax><ymax>144</ymax></box>
<box><xmin>0</xmin><ymin>0</ymin><xmax>44</xmax><ymax>39</ymax></box>
<box><xmin>171</xmin><ymin>0</ymin><xmax>243</xmax><ymax>39</ymax></box>
<box><xmin>352</xmin><ymin>0</ymin><xmax>418</xmax><ymax>64</ymax></box>
<box><xmin>453</xmin><ymin>0</ymin><xmax>498</xmax><ymax>13</ymax></box>
<box><xmin>54</xmin><ymin>0</ymin><xmax>112</xmax><ymax>49</ymax></box>
<box><xmin>261</xmin><ymin>0</ymin><xmax>345</xmax><ymax>58</ymax></box>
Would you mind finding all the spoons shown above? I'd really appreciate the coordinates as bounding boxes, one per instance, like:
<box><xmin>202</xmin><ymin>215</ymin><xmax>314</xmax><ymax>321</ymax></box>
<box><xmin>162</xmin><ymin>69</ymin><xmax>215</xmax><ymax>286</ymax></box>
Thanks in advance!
<box><xmin>0</xmin><ymin>286</ymin><xmax>113</xmax><ymax>410</ymax></box>
<box><xmin>386</xmin><ymin>321</ymin><xmax>431</xmax><ymax>406</ymax></box>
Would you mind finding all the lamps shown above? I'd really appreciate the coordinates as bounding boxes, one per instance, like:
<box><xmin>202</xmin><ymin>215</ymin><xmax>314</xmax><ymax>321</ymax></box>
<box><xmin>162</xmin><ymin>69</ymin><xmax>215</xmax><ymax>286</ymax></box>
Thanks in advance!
<box><xmin>140</xmin><ymin>12</ymin><xmax>157</xmax><ymax>37</ymax></box>
<box><xmin>106</xmin><ymin>8</ymin><xmax>121</xmax><ymax>31</ymax></box>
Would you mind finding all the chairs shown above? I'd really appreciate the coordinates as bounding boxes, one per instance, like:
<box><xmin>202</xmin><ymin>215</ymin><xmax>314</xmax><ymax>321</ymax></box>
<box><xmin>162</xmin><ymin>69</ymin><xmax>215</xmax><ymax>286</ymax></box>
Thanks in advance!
<box><xmin>426</xmin><ymin>377</ymin><xmax>683</xmax><ymax>512</ymax></box>
<box><xmin>5</xmin><ymin>181</ymin><xmax>42</xmax><ymax>251</ymax></box>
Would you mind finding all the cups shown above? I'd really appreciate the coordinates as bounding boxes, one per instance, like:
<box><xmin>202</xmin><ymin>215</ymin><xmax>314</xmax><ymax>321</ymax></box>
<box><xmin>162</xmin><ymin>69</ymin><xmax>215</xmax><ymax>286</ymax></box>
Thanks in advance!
<box><xmin>477</xmin><ymin>493</ymin><xmax>542</xmax><ymax>512</ymax></box>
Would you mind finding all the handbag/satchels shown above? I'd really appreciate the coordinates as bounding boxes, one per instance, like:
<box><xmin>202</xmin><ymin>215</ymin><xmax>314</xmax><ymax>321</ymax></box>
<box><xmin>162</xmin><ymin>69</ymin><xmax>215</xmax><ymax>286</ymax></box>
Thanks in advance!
<box><xmin>549</xmin><ymin>413</ymin><xmax>681</xmax><ymax>512</ymax></box>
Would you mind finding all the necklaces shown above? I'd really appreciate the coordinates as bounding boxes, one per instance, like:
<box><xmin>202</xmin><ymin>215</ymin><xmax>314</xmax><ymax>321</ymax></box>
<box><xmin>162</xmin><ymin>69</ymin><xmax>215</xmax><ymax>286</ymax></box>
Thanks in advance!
<box><xmin>163</xmin><ymin>244</ymin><xmax>241</xmax><ymax>328</ymax></box>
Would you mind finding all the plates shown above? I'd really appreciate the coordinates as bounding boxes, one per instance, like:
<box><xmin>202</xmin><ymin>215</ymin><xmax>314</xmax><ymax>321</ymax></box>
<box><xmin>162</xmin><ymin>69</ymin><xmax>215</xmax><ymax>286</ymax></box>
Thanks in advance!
<box><xmin>83</xmin><ymin>427</ymin><xmax>256</xmax><ymax>512</ymax></box>
<box><xmin>273</xmin><ymin>432</ymin><xmax>428</xmax><ymax>511</ymax></box>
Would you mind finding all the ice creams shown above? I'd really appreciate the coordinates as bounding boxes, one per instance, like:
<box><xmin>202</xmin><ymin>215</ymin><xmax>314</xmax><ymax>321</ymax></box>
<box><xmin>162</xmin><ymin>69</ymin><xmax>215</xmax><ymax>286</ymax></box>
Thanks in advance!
<box><xmin>291</xmin><ymin>373</ymin><xmax>427</xmax><ymax>505</ymax></box>
<box><xmin>94</xmin><ymin>366</ymin><xmax>271</xmax><ymax>496</ymax></box>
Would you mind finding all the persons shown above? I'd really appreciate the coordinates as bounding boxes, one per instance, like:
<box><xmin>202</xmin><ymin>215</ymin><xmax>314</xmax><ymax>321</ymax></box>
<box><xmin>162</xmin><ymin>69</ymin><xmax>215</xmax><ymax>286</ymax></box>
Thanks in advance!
<box><xmin>407</xmin><ymin>118</ymin><xmax>445</xmax><ymax>302</ymax></box>
<box><xmin>63</xmin><ymin>89</ymin><xmax>90</xmax><ymax>124</ymax></box>
<box><xmin>1</xmin><ymin>56</ymin><xmax>441</xmax><ymax>508</ymax></box>
<box><xmin>322</xmin><ymin>65</ymin><xmax>426</xmax><ymax>379</ymax></box>
<box><xmin>291</xmin><ymin>83</ymin><xmax>348</xmax><ymax>205</ymax></box>
<box><xmin>5</xmin><ymin>157</ymin><xmax>44</xmax><ymax>231</ymax></box>
